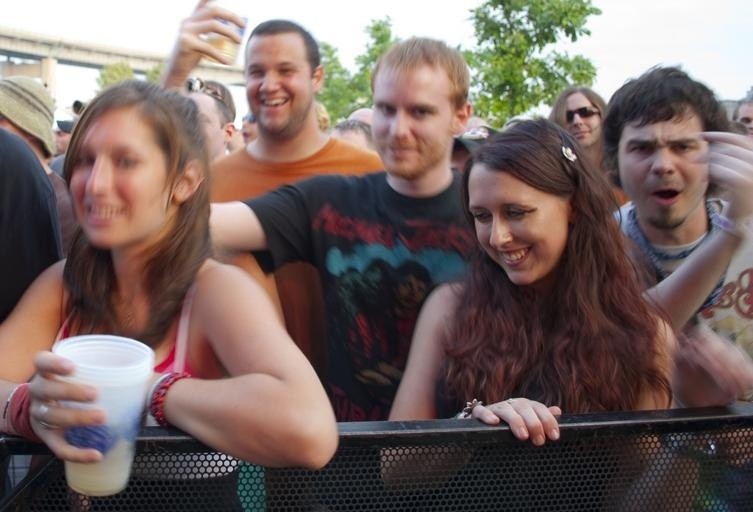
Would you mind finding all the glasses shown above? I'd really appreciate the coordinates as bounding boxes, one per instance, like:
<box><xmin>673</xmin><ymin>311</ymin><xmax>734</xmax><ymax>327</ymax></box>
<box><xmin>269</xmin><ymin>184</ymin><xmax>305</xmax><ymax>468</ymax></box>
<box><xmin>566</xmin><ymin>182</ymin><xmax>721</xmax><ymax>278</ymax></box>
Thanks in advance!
<box><xmin>566</xmin><ymin>107</ymin><xmax>599</xmax><ymax>121</ymax></box>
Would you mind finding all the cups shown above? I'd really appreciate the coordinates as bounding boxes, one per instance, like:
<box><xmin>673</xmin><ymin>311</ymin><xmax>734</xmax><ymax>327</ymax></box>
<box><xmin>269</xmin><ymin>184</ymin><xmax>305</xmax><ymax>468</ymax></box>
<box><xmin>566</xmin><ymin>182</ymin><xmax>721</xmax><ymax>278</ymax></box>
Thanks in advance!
<box><xmin>200</xmin><ymin>0</ymin><xmax>249</xmax><ymax>65</ymax></box>
<box><xmin>49</xmin><ymin>336</ymin><xmax>154</xmax><ymax>496</ymax></box>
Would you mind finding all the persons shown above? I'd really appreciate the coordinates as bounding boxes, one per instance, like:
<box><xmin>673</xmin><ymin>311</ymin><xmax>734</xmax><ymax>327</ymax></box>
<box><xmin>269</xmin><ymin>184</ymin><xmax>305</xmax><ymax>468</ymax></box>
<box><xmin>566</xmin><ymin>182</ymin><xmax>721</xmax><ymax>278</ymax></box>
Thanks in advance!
<box><xmin>378</xmin><ymin>67</ymin><xmax>752</xmax><ymax>512</ymax></box>
<box><xmin>158</xmin><ymin>0</ymin><xmax>481</xmax><ymax>512</ymax></box>
<box><xmin>0</xmin><ymin>0</ymin><xmax>338</xmax><ymax>510</ymax></box>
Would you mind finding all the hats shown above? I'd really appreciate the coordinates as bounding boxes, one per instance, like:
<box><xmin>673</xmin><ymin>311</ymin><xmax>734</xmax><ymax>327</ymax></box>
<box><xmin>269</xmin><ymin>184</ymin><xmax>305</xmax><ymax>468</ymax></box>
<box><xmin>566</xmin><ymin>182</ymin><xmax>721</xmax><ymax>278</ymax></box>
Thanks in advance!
<box><xmin>0</xmin><ymin>75</ymin><xmax>56</xmax><ymax>157</ymax></box>
